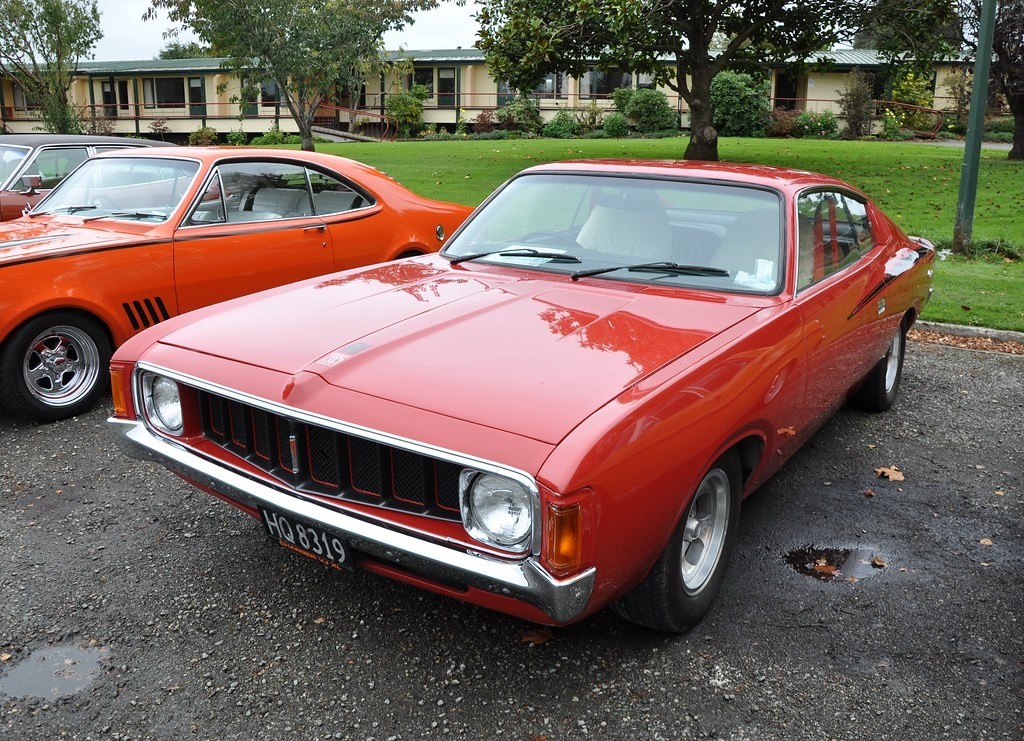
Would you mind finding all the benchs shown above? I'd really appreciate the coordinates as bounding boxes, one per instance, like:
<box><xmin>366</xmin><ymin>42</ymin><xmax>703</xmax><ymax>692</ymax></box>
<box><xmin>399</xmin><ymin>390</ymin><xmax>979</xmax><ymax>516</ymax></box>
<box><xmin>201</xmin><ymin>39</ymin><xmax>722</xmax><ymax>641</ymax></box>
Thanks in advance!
<box><xmin>237</xmin><ymin>187</ymin><xmax>371</xmax><ymax>218</ymax></box>
<box><xmin>668</xmin><ymin>220</ymin><xmax>858</xmax><ymax>273</ymax></box>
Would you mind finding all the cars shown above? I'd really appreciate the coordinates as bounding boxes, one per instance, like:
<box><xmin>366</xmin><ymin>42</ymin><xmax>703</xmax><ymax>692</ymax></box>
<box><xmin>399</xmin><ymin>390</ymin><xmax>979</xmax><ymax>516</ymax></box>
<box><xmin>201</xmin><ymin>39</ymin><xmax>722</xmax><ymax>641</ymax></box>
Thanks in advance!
<box><xmin>1</xmin><ymin>133</ymin><xmax>235</xmax><ymax>222</ymax></box>
<box><xmin>105</xmin><ymin>159</ymin><xmax>937</xmax><ymax>636</ymax></box>
<box><xmin>0</xmin><ymin>147</ymin><xmax>484</xmax><ymax>424</ymax></box>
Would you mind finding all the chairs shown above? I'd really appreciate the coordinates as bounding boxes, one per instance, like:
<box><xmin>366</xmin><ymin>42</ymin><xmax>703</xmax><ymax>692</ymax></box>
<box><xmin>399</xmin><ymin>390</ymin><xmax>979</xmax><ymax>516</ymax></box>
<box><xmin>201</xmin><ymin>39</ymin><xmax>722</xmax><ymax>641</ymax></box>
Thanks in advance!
<box><xmin>707</xmin><ymin>208</ymin><xmax>816</xmax><ymax>290</ymax></box>
<box><xmin>574</xmin><ymin>199</ymin><xmax>671</xmax><ymax>264</ymax></box>
